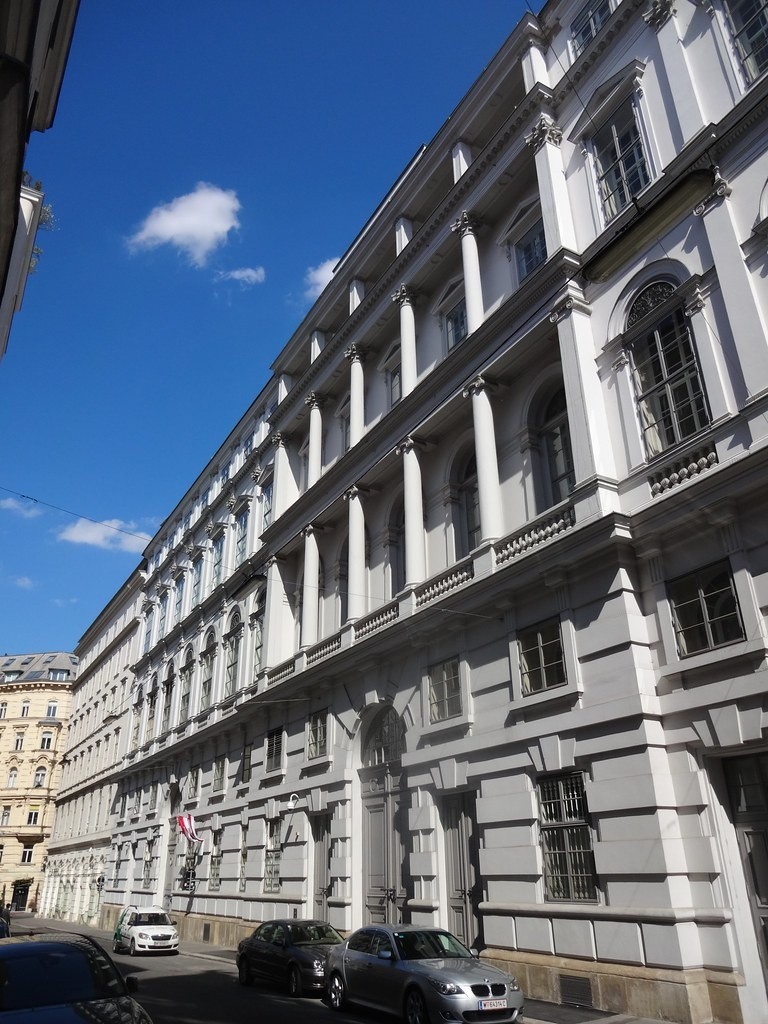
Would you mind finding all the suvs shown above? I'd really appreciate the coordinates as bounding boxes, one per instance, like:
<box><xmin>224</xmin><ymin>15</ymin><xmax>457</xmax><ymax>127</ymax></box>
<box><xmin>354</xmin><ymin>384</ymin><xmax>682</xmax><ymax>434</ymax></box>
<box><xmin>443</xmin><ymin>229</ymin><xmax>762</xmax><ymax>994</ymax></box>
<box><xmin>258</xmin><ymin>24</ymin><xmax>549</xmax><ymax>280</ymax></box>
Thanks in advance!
<box><xmin>112</xmin><ymin>904</ymin><xmax>179</xmax><ymax>955</ymax></box>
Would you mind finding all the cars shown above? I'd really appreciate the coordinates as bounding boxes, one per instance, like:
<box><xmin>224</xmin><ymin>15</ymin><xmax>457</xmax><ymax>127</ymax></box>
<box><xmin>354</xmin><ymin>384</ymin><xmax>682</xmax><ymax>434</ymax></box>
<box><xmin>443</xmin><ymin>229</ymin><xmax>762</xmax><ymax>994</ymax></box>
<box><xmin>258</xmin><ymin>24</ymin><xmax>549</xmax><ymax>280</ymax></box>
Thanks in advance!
<box><xmin>321</xmin><ymin>923</ymin><xmax>526</xmax><ymax>1024</ymax></box>
<box><xmin>0</xmin><ymin>929</ymin><xmax>154</xmax><ymax>1024</ymax></box>
<box><xmin>236</xmin><ymin>918</ymin><xmax>347</xmax><ymax>998</ymax></box>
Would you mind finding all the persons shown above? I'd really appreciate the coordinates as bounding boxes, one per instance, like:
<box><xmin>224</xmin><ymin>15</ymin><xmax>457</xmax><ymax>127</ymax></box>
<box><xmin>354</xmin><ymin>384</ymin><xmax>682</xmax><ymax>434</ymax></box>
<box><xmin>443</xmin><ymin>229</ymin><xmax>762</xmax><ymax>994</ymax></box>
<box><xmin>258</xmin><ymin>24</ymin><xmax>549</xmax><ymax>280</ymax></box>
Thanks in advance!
<box><xmin>0</xmin><ymin>905</ymin><xmax>11</xmax><ymax>938</ymax></box>
